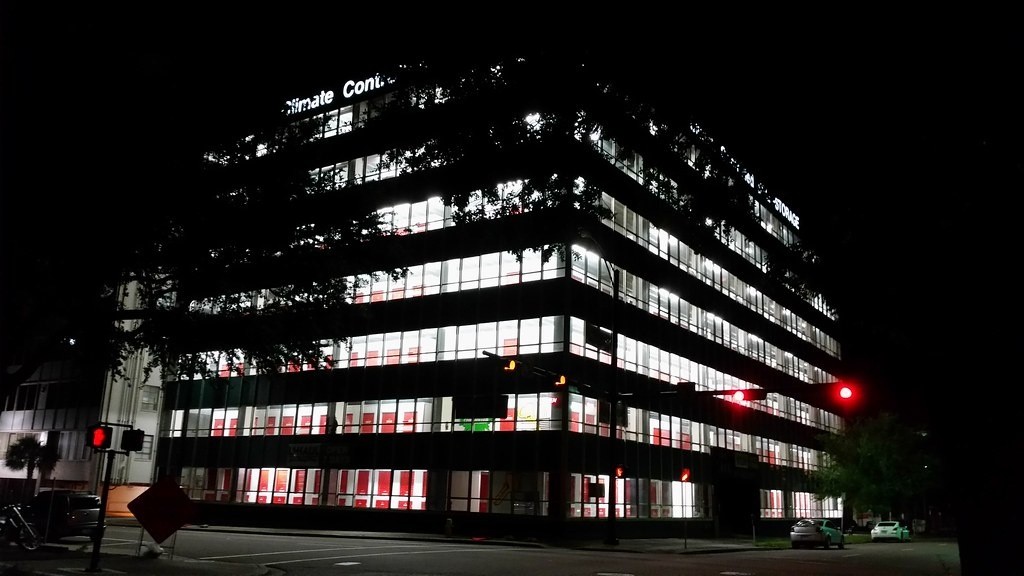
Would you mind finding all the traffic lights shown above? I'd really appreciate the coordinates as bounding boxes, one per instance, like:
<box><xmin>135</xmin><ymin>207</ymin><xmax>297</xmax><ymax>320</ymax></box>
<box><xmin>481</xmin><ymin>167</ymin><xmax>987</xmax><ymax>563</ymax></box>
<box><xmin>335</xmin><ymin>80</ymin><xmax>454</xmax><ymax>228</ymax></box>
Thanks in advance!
<box><xmin>679</xmin><ymin>468</ymin><xmax>691</xmax><ymax>483</ymax></box>
<box><xmin>499</xmin><ymin>355</ymin><xmax>522</xmax><ymax>377</ymax></box>
<box><xmin>617</xmin><ymin>464</ymin><xmax>630</xmax><ymax>481</ymax></box>
<box><xmin>85</xmin><ymin>425</ymin><xmax>113</xmax><ymax>449</ymax></box>
<box><xmin>551</xmin><ymin>370</ymin><xmax>568</xmax><ymax>391</ymax></box>
<box><xmin>728</xmin><ymin>390</ymin><xmax>762</xmax><ymax>402</ymax></box>
<box><xmin>829</xmin><ymin>381</ymin><xmax>861</xmax><ymax>403</ymax></box>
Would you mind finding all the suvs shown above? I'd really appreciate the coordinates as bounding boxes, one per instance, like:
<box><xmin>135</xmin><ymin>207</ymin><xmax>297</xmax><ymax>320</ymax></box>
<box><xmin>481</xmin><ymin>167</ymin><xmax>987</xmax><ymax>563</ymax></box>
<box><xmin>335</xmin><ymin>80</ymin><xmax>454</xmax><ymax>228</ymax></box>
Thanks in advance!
<box><xmin>31</xmin><ymin>489</ymin><xmax>108</xmax><ymax>544</ymax></box>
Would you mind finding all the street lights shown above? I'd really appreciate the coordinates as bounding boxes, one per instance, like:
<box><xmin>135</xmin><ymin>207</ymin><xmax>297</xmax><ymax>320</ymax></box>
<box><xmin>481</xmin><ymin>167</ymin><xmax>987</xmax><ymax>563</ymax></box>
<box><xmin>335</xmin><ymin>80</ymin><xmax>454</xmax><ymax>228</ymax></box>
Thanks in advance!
<box><xmin>577</xmin><ymin>226</ymin><xmax>621</xmax><ymax>545</ymax></box>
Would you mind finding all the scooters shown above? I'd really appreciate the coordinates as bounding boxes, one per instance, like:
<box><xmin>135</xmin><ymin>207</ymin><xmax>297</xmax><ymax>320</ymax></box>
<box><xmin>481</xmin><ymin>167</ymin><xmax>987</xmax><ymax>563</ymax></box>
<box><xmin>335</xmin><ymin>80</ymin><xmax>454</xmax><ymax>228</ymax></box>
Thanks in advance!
<box><xmin>0</xmin><ymin>498</ymin><xmax>46</xmax><ymax>556</ymax></box>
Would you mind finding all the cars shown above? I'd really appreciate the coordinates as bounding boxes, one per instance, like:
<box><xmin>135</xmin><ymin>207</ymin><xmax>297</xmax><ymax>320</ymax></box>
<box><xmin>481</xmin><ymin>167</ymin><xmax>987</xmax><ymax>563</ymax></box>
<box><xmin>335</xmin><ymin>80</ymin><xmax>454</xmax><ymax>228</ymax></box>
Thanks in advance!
<box><xmin>848</xmin><ymin>508</ymin><xmax>958</xmax><ymax>543</ymax></box>
<box><xmin>789</xmin><ymin>518</ymin><xmax>845</xmax><ymax>551</ymax></box>
